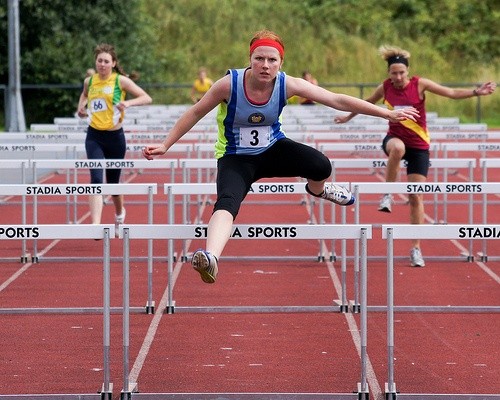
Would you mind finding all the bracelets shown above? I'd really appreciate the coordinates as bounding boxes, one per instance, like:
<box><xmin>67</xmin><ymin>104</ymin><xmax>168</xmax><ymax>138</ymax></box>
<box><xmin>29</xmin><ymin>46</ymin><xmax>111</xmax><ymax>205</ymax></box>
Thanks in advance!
<box><xmin>473</xmin><ymin>88</ymin><xmax>480</xmax><ymax>96</ymax></box>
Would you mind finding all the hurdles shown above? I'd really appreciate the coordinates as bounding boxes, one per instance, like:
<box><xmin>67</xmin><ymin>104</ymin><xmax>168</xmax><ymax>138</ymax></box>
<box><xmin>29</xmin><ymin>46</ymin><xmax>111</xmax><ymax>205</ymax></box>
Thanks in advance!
<box><xmin>117</xmin><ymin>223</ymin><xmax>374</xmax><ymax>400</ymax></box>
<box><xmin>0</xmin><ymin>182</ymin><xmax>159</xmax><ymax>316</ymax></box>
<box><xmin>381</xmin><ymin>222</ymin><xmax>500</xmax><ymax>400</ymax></box>
<box><xmin>162</xmin><ymin>180</ymin><xmax>351</xmax><ymax>315</ymax></box>
<box><xmin>0</xmin><ymin>222</ymin><xmax>117</xmax><ymax>400</ymax></box>
<box><xmin>0</xmin><ymin>95</ymin><xmax>500</xmax><ymax>209</ymax></box>
<box><xmin>349</xmin><ymin>180</ymin><xmax>500</xmax><ymax>316</ymax></box>
<box><xmin>0</xmin><ymin>157</ymin><xmax>180</xmax><ymax>265</ymax></box>
<box><xmin>178</xmin><ymin>157</ymin><xmax>332</xmax><ymax>265</ymax></box>
<box><xmin>330</xmin><ymin>157</ymin><xmax>500</xmax><ymax>263</ymax></box>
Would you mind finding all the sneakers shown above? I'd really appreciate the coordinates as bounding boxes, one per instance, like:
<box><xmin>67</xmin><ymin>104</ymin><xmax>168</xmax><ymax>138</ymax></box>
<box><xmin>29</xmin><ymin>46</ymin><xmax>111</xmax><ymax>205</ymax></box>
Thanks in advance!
<box><xmin>377</xmin><ymin>195</ymin><xmax>392</xmax><ymax>213</ymax></box>
<box><xmin>191</xmin><ymin>248</ymin><xmax>218</xmax><ymax>283</ymax></box>
<box><xmin>409</xmin><ymin>247</ymin><xmax>426</xmax><ymax>266</ymax></box>
<box><xmin>305</xmin><ymin>181</ymin><xmax>355</xmax><ymax>206</ymax></box>
<box><xmin>114</xmin><ymin>205</ymin><xmax>126</xmax><ymax>237</ymax></box>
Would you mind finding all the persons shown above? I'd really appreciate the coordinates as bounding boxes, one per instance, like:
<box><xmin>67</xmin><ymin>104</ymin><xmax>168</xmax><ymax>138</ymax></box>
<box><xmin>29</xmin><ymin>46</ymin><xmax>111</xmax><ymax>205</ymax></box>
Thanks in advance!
<box><xmin>142</xmin><ymin>30</ymin><xmax>421</xmax><ymax>284</ymax></box>
<box><xmin>297</xmin><ymin>70</ymin><xmax>318</xmax><ymax>105</ymax></box>
<box><xmin>190</xmin><ymin>68</ymin><xmax>214</xmax><ymax>105</ymax></box>
<box><xmin>76</xmin><ymin>43</ymin><xmax>153</xmax><ymax>242</ymax></box>
<box><xmin>333</xmin><ymin>45</ymin><xmax>497</xmax><ymax>266</ymax></box>
